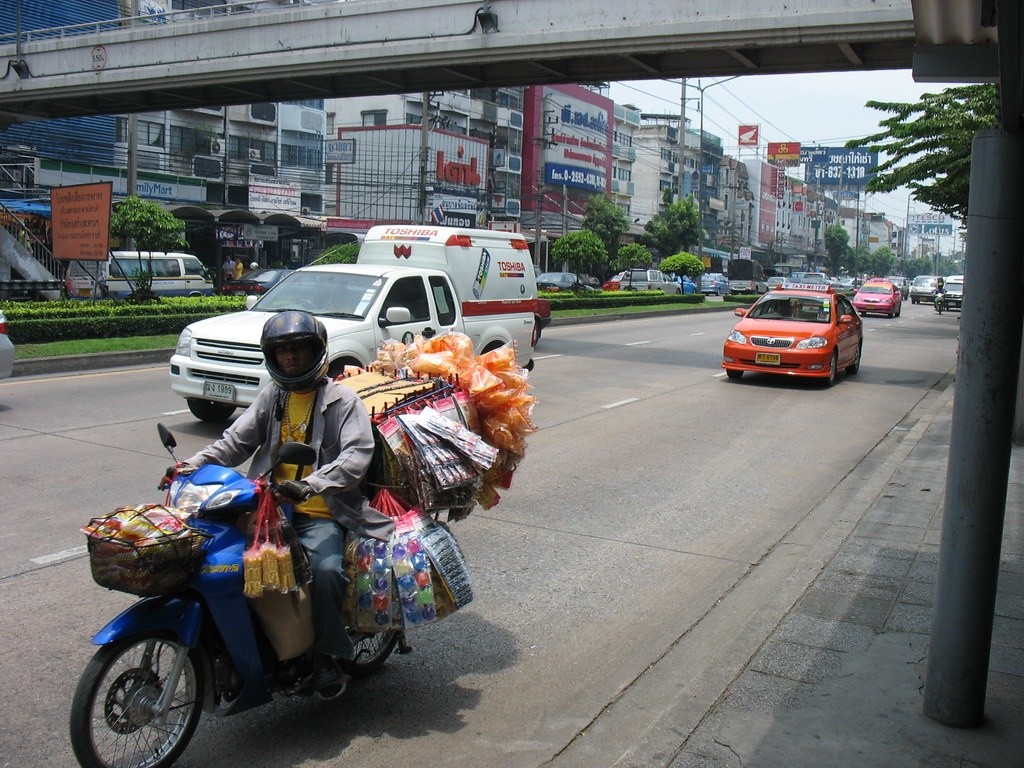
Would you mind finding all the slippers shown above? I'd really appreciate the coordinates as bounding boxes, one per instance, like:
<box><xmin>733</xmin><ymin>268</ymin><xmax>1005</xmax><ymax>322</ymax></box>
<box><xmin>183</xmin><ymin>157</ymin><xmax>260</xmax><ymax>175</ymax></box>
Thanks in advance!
<box><xmin>314</xmin><ymin>653</ymin><xmax>348</xmax><ymax>700</ymax></box>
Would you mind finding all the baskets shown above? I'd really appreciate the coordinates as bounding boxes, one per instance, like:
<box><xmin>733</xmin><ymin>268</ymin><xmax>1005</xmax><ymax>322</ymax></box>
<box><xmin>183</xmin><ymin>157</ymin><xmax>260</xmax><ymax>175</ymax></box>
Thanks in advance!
<box><xmin>86</xmin><ymin>522</ymin><xmax>215</xmax><ymax>595</ymax></box>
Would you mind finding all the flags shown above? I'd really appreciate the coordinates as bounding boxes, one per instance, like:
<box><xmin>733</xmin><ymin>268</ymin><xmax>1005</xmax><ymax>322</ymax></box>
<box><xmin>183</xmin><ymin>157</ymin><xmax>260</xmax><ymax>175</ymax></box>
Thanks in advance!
<box><xmin>431</xmin><ymin>205</ymin><xmax>445</xmax><ymax>225</ymax></box>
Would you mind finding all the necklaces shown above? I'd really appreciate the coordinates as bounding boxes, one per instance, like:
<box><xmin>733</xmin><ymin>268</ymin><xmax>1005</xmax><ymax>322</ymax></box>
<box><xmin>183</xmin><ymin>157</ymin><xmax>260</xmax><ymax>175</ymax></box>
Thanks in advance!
<box><xmin>285</xmin><ymin>390</ymin><xmax>318</xmax><ymax>442</ymax></box>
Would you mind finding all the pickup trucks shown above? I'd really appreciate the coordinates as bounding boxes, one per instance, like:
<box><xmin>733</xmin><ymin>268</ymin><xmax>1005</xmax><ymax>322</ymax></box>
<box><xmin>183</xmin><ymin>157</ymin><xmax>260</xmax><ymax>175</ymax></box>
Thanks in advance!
<box><xmin>165</xmin><ymin>222</ymin><xmax>543</xmax><ymax>428</ymax></box>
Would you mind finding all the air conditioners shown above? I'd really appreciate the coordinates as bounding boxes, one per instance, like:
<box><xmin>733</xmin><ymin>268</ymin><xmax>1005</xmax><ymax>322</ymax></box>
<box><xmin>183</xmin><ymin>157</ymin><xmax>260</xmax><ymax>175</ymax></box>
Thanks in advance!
<box><xmin>210</xmin><ymin>137</ymin><xmax>226</xmax><ymax>157</ymax></box>
<box><xmin>245</xmin><ymin>148</ymin><xmax>260</xmax><ymax>159</ymax></box>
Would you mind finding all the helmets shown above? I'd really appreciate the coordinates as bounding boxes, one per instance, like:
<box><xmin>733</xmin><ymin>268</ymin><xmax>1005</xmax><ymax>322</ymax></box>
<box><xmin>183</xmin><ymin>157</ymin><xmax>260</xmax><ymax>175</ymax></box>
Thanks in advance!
<box><xmin>250</xmin><ymin>262</ymin><xmax>259</xmax><ymax>270</ymax></box>
<box><xmin>938</xmin><ymin>283</ymin><xmax>942</xmax><ymax>286</ymax></box>
<box><xmin>260</xmin><ymin>313</ymin><xmax>330</xmax><ymax>392</ymax></box>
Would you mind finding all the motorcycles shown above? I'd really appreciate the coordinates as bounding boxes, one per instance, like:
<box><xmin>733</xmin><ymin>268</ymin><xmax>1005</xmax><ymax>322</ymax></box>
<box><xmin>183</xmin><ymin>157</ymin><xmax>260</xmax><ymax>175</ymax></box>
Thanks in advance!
<box><xmin>64</xmin><ymin>425</ymin><xmax>419</xmax><ymax>768</ymax></box>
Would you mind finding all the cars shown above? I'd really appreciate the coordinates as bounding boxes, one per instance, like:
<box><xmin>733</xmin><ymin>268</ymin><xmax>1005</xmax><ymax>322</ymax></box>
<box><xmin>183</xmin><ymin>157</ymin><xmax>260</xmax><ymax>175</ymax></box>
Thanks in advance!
<box><xmin>720</xmin><ymin>282</ymin><xmax>864</xmax><ymax>388</ymax></box>
<box><xmin>852</xmin><ymin>276</ymin><xmax>902</xmax><ymax>319</ymax></box>
<box><xmin>599</xmin><ymin>257</ymin><xmax>966</xmax><ymax>318</ymax></box>
<box><xmin>219</xmin><ymin>267</ymin><xmax>296</xmax><ymax>299</ymax></box>
<box><xmin>535</xmin><ymin>271</ymin><xmax>595</xmax><ymax>293</ymax></box>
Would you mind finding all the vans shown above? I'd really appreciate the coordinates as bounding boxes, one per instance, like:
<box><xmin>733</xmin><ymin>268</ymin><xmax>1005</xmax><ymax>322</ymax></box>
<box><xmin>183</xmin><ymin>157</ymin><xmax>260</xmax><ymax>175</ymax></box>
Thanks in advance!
<box><xmin>95</xmin><ymin>248</ymin><xmax>216</xmax><ymax>301</ymax></box>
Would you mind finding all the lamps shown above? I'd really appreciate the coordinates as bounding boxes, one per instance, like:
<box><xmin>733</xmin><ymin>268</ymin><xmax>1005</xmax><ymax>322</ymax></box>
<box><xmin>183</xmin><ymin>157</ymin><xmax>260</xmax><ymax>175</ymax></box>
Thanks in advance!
<box><xmin>11</xmin><ymin>63</ymin><xmax>28</xmax><ymax>80</ymax></box>
<box><xmin>478</xmin><ymin>11</ymin><xmax>498</xmax><ymax>32</ymax></box>
<box><xmin>302</xmin><ymin>207</ymin><xmax>311</xmax><ymax>215</ymax></box>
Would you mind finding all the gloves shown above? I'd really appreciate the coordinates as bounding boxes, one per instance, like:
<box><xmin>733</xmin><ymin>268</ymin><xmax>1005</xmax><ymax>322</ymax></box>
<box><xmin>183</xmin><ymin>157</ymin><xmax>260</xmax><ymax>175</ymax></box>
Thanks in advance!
<box><xmin>276</xmin><ymin>480</ymin><xmax>313</xmax><ymax>502</ymax></box>
<box><xmin>160</xmin><ymin>463</ymin><xmax>190</xmax><ymax>491</ymax></box>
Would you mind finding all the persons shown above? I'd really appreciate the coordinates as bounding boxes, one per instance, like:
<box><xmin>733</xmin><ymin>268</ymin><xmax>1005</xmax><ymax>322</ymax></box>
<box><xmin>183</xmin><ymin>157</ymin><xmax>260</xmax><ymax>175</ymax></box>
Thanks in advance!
<box><xmin>934</xmin><ymin>283</ymin><xmax>945</xmax><ymax>295</ymax></box>
<box><xmin>223</xmin><ymin>255</ymin><xmax>243</xmax><ymax>279</ymax></box>
<box><xmin>160</xmin><ymin>310</ymin><xmax>375</xmax><ymax>701</ymax></box>
